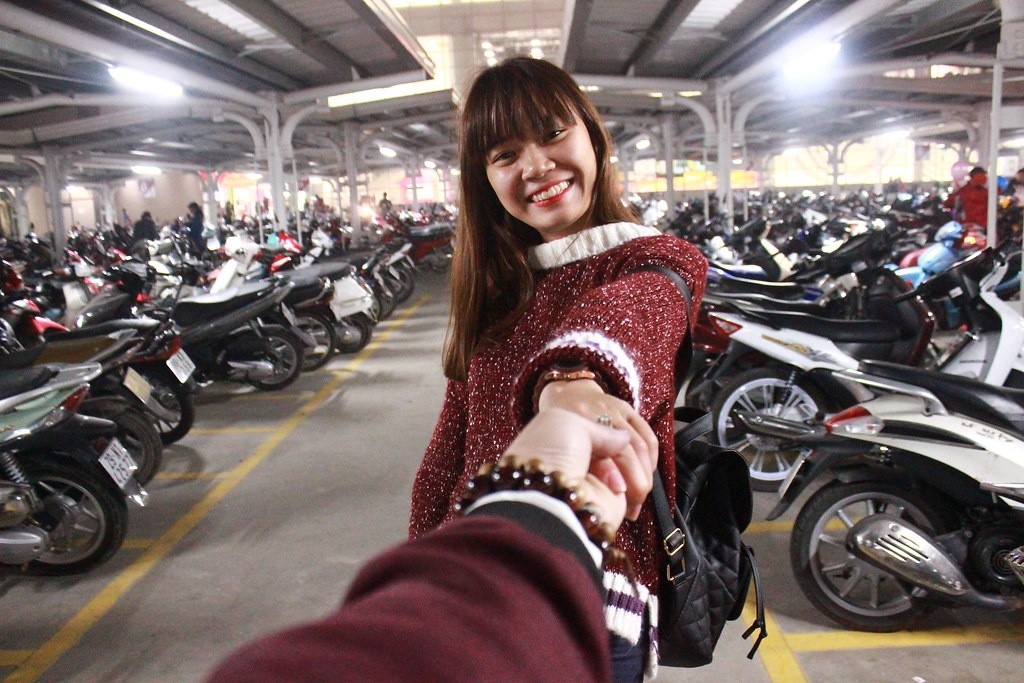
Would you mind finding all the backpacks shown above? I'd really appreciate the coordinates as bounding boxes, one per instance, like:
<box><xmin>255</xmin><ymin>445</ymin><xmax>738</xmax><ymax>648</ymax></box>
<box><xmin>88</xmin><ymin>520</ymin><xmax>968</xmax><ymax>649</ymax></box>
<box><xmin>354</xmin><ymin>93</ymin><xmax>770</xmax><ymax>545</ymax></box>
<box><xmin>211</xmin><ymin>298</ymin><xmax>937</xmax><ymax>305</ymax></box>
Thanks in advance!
<box><xmin>627</xmin><ymin>265</ymin><xmax>767</xmax><ymax>669</ymax></box>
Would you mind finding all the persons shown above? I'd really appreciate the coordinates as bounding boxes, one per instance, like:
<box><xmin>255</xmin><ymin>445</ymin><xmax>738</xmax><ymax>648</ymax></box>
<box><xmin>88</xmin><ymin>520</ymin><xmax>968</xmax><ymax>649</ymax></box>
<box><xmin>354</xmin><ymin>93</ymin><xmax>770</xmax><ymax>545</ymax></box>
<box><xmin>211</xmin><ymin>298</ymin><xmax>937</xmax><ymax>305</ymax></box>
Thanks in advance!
<box><xmin>408</xmin><ymin>58</ymin><xmax>709</xmax><ymax>683</ymax></box>
<box><xmin>946</xmin><ymin>167</ymin><xmax>989</xmax><ymax>241</ymax></box>
<box><xmin>131</xmin><ymin>188</ymin><xmax>394</xmax><ymax>262</ymax></box>
<box><xmin>1005</xmin><ymin>169</ymin><xmax>1024</xmax><ymax>210</ymax></box>
<box><xmin>191</xmin><ymin>410</ymin><xmax>627</xmax><ymax>683</ymax></box>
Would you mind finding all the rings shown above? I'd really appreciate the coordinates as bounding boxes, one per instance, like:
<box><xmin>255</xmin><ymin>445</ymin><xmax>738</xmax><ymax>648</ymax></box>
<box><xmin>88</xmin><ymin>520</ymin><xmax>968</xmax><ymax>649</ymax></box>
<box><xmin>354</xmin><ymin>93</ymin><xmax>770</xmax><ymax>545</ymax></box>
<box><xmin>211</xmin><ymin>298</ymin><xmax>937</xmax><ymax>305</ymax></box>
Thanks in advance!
<box><xmin>596</xmin><ymin>415</ymin><xmax>612</xmax><ymax>428</ymax></box>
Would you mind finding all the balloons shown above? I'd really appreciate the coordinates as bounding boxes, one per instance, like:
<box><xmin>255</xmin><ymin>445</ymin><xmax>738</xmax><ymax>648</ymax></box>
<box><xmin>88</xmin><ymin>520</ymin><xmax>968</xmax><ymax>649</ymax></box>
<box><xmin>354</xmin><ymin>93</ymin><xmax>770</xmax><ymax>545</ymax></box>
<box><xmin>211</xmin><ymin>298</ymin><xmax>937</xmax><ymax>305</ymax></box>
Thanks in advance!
<box><xmin>952</xmin><ymin>160</ymin><xmax>969</xmax><ymax>182</ymax></box>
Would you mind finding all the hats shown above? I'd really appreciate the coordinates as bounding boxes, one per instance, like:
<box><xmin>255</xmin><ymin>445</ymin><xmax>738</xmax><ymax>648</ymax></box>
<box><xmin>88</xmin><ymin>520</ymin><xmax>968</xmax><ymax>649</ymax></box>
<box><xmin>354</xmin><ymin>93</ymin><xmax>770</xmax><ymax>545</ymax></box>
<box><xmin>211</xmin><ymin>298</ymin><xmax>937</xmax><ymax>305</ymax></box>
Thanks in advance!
<box><xmin>969</xmin><ymin>167</ymin><xmax>986</xmax><ymax>176</ymax></box>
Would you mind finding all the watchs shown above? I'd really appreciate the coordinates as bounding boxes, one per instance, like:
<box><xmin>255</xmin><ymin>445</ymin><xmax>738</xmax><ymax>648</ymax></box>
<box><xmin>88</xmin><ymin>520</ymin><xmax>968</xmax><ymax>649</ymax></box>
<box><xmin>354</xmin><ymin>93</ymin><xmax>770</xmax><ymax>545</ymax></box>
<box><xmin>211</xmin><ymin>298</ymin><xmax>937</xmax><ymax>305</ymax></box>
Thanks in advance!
<box><xmin>530</xmin><ymin>359</ymin><xmax>614</xmax><ymax>413</ymax></box>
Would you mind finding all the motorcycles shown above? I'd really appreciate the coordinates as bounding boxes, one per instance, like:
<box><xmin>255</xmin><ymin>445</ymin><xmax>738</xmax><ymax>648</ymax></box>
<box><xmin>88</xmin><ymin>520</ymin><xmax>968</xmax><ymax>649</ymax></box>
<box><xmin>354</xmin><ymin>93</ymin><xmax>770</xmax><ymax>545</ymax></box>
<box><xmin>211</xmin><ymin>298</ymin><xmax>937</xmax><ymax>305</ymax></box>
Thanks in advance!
<box><xmin>635</xmin><ymin>179</ymin><xmax>1024</xmax><ymax>632</ymax></box>
<box><xmin>0</xmin><ymin>189</ymin><xmax>464</xmax><ymax>579</ymax></box>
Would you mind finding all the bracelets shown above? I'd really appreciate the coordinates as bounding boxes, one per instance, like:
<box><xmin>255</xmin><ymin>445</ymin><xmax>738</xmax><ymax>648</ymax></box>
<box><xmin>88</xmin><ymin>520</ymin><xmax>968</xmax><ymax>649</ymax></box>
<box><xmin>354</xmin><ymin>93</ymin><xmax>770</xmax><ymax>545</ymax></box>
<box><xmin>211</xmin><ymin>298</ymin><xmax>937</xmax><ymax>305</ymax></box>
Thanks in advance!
<box><xmin>450</xmin><ymin>452</ymin><xmax>639</xmax><ymax>600</ymax></box>
